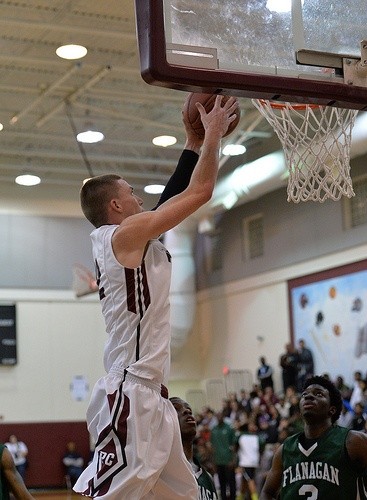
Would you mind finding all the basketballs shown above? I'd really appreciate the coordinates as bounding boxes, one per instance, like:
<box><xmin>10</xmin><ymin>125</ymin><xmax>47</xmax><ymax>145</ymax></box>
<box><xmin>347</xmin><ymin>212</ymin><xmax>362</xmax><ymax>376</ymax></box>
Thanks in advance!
<box><xmin>183</xmin><ymin>91</ymin><xmax>240</xmax><ymax>139</ymax></box>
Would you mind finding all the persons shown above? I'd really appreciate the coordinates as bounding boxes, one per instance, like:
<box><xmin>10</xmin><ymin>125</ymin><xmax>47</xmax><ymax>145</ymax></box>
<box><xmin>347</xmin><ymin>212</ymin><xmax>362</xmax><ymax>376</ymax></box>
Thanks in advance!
<box><xmin>169</xmin><ymin>396</ymin><xmax>219</xmax><ymax>500</ymax></box>
<box><xmin>0</xmin><ymin>434</ymin><xmax>34</xmax><ymax>500</ymax></box>
<box><xmin>62</xmin><ymin>440</ymin><xmax>83</xmax><ymax>483</ymax></box>
<box><xmin>170</xmin><ymin>338</ymin><xmax>367</xmax><ymax>500</ymax></box>
<box><xmin>259</xmin><ymin>376</ymin><xmax>367</xmax><ymax>500</ymax></box>
<box><xmin>79</xmin><ymin>94</ymin><xmax>242</xmax><ymax>500</ymax></box>
